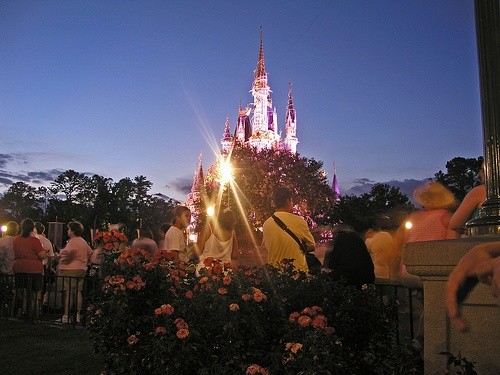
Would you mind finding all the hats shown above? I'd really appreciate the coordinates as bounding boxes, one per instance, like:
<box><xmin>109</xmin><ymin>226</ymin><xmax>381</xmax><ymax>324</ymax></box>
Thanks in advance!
<box><xmin>413</xmin><ymin>182</ymin><xmax>454</xmax><ymax>208</ymax></box>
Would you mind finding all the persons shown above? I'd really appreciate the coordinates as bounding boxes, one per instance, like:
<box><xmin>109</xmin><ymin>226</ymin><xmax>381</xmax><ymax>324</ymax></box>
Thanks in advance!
<box><xmin>328</xmin><ymin>213</ymin><xmax>394</xmax><ymax>286</ymax></box>
<box><xmin>262</xmin><ymin>187</ymin><xmax>316</xmax><ymax>278</ymax></box>
<box><xmin>448</xmin><ymin>162</ymin><xmax>485</xmax><ymax>239</ymax></box>
<box><xmin>0</xmin><ymin>189</ymin><xmax>237</xmax><ymax>327</ymax></box>
<box><xmin>445</xmin><ymin>241</ymin><xmax>500</xmax><ymax>330</ymax></box>
<box><xmin>396</xmin><ymin>183</ymin><xmax>461</xmax><ymax>280</ymax></box>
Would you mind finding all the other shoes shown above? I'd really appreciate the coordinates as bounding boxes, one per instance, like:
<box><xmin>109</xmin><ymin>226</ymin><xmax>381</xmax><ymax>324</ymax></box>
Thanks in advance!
<box><xmin>55</xmin><ymin>314</ymin><xmax>71</xmax><ymax>323</ymax></box>
<box><xmin>76</xmin><ymin>313</ymin><xmax>81</xmax><ymax>323</ymax></box>
<box><xmin>38</xmin><ymin>295</ymin><xmax>48</xmax><ymax>303</ymax></box>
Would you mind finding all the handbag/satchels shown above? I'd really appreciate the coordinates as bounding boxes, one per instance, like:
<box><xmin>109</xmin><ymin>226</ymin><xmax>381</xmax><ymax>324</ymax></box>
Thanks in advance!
<box><xmin>304</xmin><ymin>252</ymin><xmax>322</xmax><ymax>276</ymax></box>
